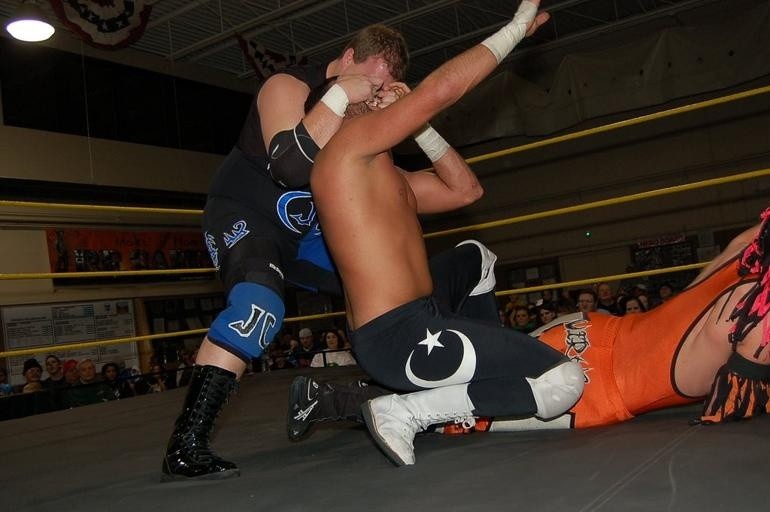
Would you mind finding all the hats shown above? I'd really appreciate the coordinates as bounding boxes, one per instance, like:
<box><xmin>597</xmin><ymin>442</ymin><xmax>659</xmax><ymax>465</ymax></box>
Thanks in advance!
<box><xmin>530</xmin><ymin>298</ymin><xmax>554</xmax><ymax>313</ymax></box>
<box><xmin>23</xmin><ymin>358</ymin><xmax>43</xmax><ymax>373</ymax></box>
<box><xmin>299</xmin><ymin>328</ymin><xmax>312</xmax><ymax>339</ymax></box>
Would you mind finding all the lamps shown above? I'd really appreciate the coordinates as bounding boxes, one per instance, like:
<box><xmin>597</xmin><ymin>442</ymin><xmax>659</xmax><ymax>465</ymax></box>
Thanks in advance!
<box><xmin>5</xmin><ymin>4</ymin><xmax>56</xmax><ymax>43</ymax></box>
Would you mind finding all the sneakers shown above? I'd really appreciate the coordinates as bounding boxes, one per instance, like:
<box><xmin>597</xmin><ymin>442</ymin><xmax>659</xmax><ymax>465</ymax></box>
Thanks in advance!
<box><xmin>286</xmin><ymin>376</ymin><xmax>323</xmax><ymax>440</ymax></box>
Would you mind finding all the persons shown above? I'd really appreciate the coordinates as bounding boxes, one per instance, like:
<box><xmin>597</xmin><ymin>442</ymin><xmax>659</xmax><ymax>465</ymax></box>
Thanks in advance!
<box><xmin>302</xmin><ymin>0</ymin><xmax>586</xmax><ymax>468</ymax></box>
<box><xmin>249</xmin><ymin>322</ymin><xmax>354</xmax><ymax>372</ymax></box>
<box><xmin>160</xmin><ymin>24</ymin><xmax>414</xmax><ymax>482</ymax></box>
<box><xmin>2</xmin><ymin>348</ymin><xmax>198</xmax><ymax>405</ymax></box>
<box><xmin>492</xmin><ymin>282</ymin><xmax>670</xmax><ymax>333</ymax></box>
<box><xmin>425</xmin><ymin>209</ymin><xmax>770</xmax><ymax>432</ymax></box>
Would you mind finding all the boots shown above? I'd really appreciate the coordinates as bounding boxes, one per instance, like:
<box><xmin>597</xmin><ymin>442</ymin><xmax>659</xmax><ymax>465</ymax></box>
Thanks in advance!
<box><xmin>159</xmin><ymin>364</ymin><xmax>240</xmax><ymax>481</ymax></box>
<box><xmin>361</xmin><ymin>382</ymin><xmax>475</xmax><ymax>467</ymax></box>
